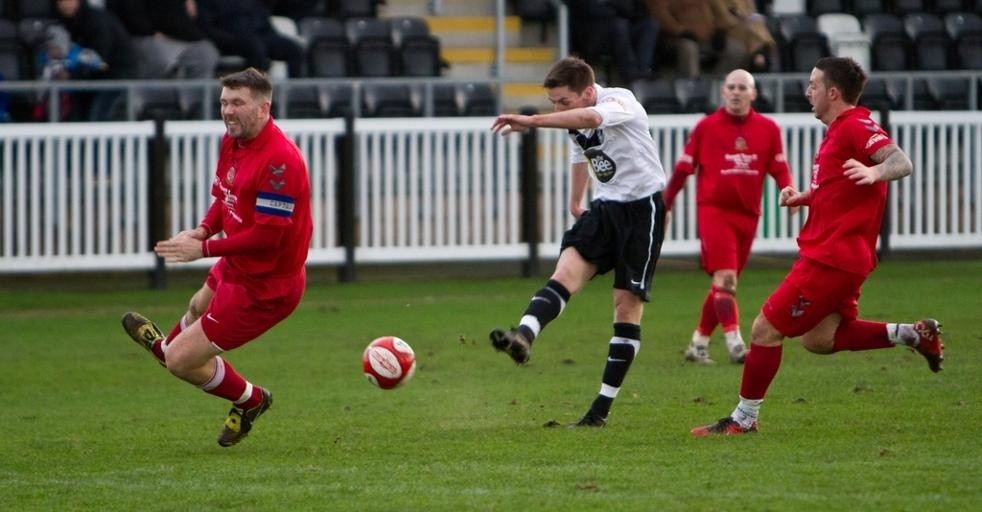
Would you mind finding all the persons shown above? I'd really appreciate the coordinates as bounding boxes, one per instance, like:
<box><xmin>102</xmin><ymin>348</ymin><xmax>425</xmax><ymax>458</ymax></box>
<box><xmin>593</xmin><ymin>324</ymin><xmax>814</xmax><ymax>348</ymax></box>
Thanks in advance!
<box><xmin>685</xmin><ymin>54</ymin><xmax>947</xmax><ymax>439</ymax></box>
<box><xmin>645</xmin><ymin>0</ymin><xmax>755</xmax><ymax>76</ymax></box>
<box><xmin>121</xmin><ymin>69</ymin><xmax>313</xmax><ymax>448</ymax></box>
<box><xmin>0</xmin><ymin>0</ymin><xmax>317</xmax><ymax>122</ymax></box>
<box><xmin>576</xmin><ymin>1</ymin><xmax>658</xmax><ymax>82</ymax></box>
<box><xmin>662</xmin><ymin>66</ymin><xmax>794</xmax><ymax>364</ymax></box>
<box><xmin>486</xmin><ymin>53</ymin><xmax>666</xmax><ymax>429</ymax></box>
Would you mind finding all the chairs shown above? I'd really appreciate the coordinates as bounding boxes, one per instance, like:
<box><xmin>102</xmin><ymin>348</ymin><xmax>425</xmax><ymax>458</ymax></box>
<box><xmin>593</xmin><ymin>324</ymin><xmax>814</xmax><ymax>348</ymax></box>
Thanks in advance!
<box><xmin>288</xmin><ymin>1</ymin><xmax>982</xmax><ymax>120</ymax></box>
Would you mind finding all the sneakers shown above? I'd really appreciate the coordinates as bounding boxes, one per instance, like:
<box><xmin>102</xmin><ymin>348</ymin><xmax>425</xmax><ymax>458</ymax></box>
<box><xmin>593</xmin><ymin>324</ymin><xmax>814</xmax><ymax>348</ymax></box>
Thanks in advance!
<box><xmin>913</xmin><ymin>318</ymin><xmax>945</xmax><ymax>372</ymax></box>
<box><xmin>121</xmin><ymin>312</ymin><xmax>170</xmax><ymax>367</ymax></box>
<box><xmin>543</xmin><ymin>416</ymin><xmax>606</xmax><ymax>430</ymax></box>
<box><xmin>728</xmin><ymin>345</ymin><xmax>749</xmax><ymax>363</ymax></box>
<box><xmin>489</xmin><ymin>327</ymin><xmax>531</xmax><ymax>365</ymax></box>
<box><xmin>218</xmin><ymin>387</ymin><xmax>273</xmax><ymax>447</ymax></box>
<box><xmin>685</xmin><ymin>344</ymin><xmax>716</xmax><ymax>365</ymax></box>
<box><xmin>689</xmin><ymin>417</ymin><xmax>758</xmax><ymax>435</ymax></box>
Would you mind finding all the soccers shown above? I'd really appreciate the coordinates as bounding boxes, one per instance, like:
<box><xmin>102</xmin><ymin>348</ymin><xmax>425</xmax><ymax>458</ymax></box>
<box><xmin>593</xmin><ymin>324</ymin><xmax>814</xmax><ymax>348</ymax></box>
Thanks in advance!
<box><xmin>362</xmin><ymin>335</ymin><xmax>416</xmax><ymax>390</ymax></box>
